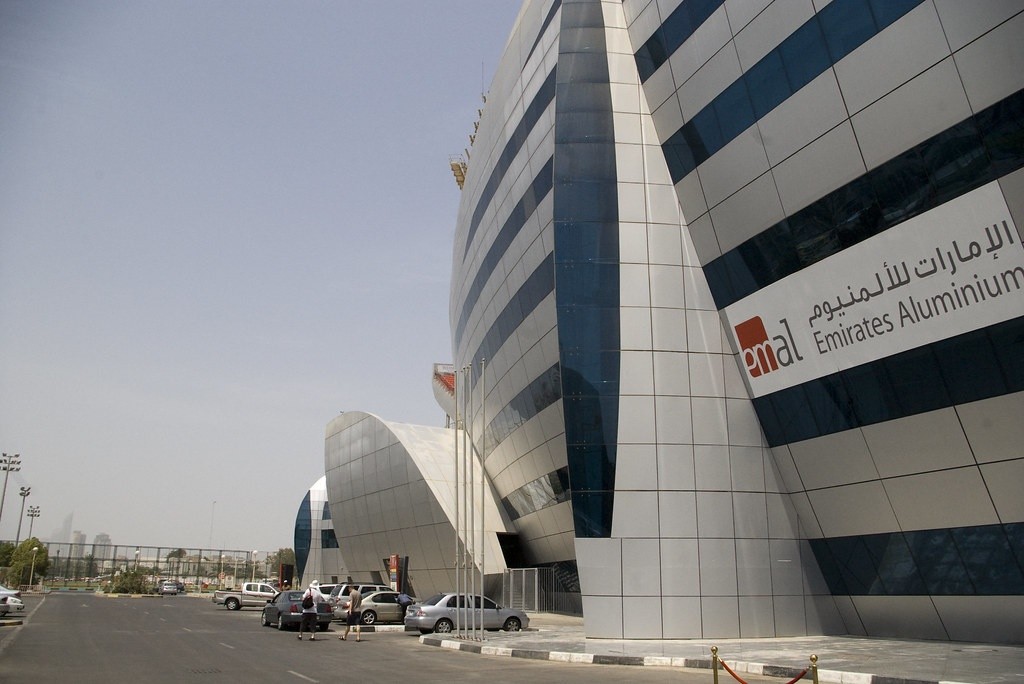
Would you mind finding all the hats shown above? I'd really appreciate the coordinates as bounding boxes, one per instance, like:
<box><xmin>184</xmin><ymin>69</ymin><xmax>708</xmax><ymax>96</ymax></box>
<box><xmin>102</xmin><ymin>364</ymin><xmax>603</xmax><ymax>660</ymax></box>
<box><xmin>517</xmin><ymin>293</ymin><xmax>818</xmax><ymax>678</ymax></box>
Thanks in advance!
<box><xmin>309</xmin><ymin>579</ymin><xmax>319</xmax><ymax>588</ymax></box>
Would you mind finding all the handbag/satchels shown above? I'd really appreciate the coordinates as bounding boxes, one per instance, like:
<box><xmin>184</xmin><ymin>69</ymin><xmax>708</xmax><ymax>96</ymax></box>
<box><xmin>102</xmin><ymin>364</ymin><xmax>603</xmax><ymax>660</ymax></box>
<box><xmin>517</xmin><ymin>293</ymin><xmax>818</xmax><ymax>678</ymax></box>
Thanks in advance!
<box><xmin>302</xmin><ymin>588</ymin><xmax>314</xmax><ymax>609</ymax></box>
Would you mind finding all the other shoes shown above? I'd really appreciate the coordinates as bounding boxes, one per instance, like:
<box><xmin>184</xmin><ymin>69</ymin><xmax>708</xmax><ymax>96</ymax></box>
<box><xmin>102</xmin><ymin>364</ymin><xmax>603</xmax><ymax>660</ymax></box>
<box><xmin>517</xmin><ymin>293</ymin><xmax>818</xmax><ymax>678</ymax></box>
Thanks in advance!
<box><xmin>308</xmin><ymin>636</ymin><xmax>315</xmax><ymax>641</ymax></box>
<box><xmin>298</xmin><ymin>634</ymin><xmax>302</xmax><ymax>640</ymax></box>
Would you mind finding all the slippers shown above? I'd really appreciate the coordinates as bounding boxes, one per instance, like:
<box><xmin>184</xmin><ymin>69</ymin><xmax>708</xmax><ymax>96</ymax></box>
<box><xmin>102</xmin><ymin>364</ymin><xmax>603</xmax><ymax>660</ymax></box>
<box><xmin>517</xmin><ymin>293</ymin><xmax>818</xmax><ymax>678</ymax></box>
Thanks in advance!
<box><xmin>352</xmin><ymin>639</ymin><xmax>360</xmax><ymax>642</ymax></box>
<box><xmin>338</xmin><ymin>635</ymin><xmax>346</xmax><ymax>641</ymax></box>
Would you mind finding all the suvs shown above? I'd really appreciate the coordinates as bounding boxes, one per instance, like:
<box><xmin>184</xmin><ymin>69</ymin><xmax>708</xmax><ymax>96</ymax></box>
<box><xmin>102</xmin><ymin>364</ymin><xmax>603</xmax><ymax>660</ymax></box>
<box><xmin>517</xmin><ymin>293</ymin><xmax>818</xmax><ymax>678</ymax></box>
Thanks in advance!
<box><xmin>315</xmin><ymin>583</ymin><xmax>341</xmax><ymax>602</ymax></box>
<box><xmin>328</xmin><ymin>582</ymin><xmax>397</xmax><ymax>622</ymax></box>
<box><xmin>154</xmin><ymin>582</ymin><xmax>184</xmax><ymax>595</ymax></box>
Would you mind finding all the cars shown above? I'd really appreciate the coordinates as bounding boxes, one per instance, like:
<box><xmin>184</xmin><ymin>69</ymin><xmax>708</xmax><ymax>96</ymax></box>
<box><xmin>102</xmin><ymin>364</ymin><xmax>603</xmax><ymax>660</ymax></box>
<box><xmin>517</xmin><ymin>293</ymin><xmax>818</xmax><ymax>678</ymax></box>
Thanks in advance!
<box><xmin>260</xmin><ymin>591</ymin><xmax>333</xmax><ymax>632</ymax></box>
<box><xmin>405</xmin><ymin>592</ymin><xmax>530</xmax><ymax>636</ymax></box>
<box><xmin>335</xmin><ymin>591</ymin><xmax>420</xmax><ymax>625</ymax></box>
<box><xmin>0</xmin><ymin>586</ymin><xmax>22</xmax><ymax>601</ymax></box>
<box><xmin>161</xmin><ymin>582</ymin><xmax>177</xmax><ymax>595</ymax></box>
<box><xmin>6</xmin><ymin>596</ymin><xmax>25</xmax><ymax>613</ymax></box>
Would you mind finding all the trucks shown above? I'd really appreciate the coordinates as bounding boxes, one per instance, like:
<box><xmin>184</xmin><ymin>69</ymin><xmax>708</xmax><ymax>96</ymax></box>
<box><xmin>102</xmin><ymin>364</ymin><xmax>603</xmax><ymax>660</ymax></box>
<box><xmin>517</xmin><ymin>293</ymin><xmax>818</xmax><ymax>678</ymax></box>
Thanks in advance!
<box><xmin>212</xmin><ymin>582</ymin><xmax>280</xmax><ymax>611</ymax></box>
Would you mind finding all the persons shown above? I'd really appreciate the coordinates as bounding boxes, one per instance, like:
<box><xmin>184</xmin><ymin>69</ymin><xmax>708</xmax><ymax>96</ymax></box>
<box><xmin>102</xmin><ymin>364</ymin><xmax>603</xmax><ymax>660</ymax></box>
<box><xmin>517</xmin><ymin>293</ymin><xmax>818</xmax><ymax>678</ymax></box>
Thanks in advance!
<box><xmin>338</xmin><ymin>586</ymin><xmax>362</xmax><ymax>642</ymax></box>
<box><xmin>397</xmin><ymin>591</ymin><xmax>413</xmax><ymax>625</ymax></box>
<box><xmin>281</xmin><ymin>580</ymin><xmax>291</xmax><ymax>591</ymax></box>
<box><xmin>298</xmin><ymin>580</ymin><xmax>321</xmax><ymax>641</ymax></box>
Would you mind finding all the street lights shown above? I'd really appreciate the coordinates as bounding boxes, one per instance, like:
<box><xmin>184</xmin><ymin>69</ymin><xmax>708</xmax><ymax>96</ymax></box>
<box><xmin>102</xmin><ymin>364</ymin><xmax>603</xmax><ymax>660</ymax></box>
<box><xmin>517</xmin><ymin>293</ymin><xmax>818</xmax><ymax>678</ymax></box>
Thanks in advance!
<box><xmin>25</xmin><ymin>505</ymin><xmax>41</xmax><ymax>540</ymax></box>
<box><xmin>0</xmin><ymin>451</ymin><xmax>22</xmax><ymax>519</ymax></box>
<box><xmin>134</xmin><ymin>550</ymin><xmax>140</xmax><ymax>572</ymax></box>
<box><xmin>12</xmin><ymin>486</ymin><xmax>32</xmax><ymax>547</ymax></box>
<box><xmin>219</xmin><ymin>554</ymin><xmax>226</xmax><ymax>589</ymax></box>
<box><xmin>253</xmin><ymin>550</ymin><xmax>258</xmax><ymax>581</ymax></box>
<box><xmin>26</xmin><ymin>547</ymin><xmax>39</xmax><ymax>593</ymax></box>
